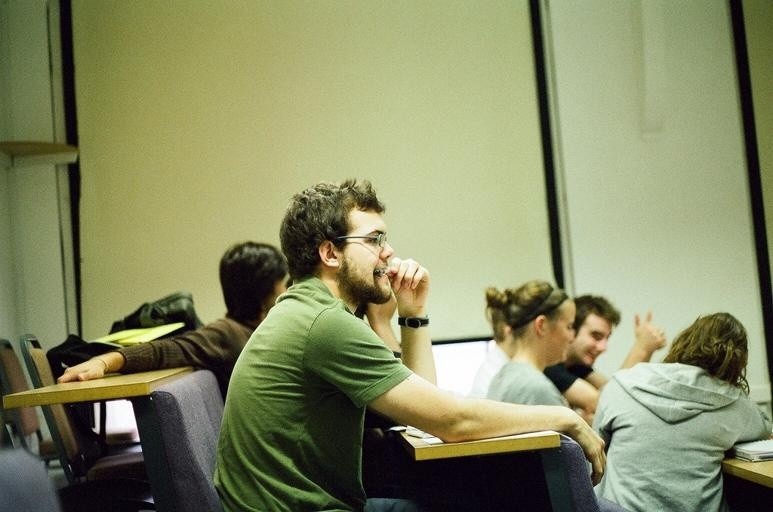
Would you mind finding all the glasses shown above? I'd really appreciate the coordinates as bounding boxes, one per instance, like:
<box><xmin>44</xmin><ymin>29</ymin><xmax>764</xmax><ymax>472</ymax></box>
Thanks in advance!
<box><xmin>337</xmin><ymin>232</ymin><xmax>387</xmax><ymax>247</ymax></box>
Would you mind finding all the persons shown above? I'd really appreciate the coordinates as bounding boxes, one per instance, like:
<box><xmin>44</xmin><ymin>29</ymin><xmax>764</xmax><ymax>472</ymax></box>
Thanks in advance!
<box><xmin>586</xmin><ymin>312</ymin><xmax>772</xmax><ymax>512</ymax></box>
<box><xmin>214</xmin><ymin>178</ymin><xmax>607</xmax><ymax>512</ymax></box>
<box><xmin>483</xmin><ymin>280</ymin><xmax>607</xmax><ymax>500</ymax></box>
<box><xmin>540</xmin><ymin>293</ymin><xmax>667</xmax><ymax>428</ymax></box>
<box><xmin>57</xmin><ymin>242</ymin><xmax>287</xmax><ymax>412</ymax></box>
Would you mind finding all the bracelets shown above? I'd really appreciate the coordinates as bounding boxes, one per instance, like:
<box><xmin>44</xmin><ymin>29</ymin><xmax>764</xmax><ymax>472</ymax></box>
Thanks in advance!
<box><xmin>397</xmin><ymin>316</ymin><xmax>429</xmax><ymax>329</ymax></box>
<box><xmin>91</xmin><ymin>357</ymin><xmax>109</xmax><ymax>374</ymax></box>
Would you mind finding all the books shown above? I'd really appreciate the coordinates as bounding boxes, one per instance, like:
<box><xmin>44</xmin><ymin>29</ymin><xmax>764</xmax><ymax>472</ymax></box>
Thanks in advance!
<box><xmin>731</xmin><ymin>439</ymin><xmax>773</xmax><ymax>463</ymax></box>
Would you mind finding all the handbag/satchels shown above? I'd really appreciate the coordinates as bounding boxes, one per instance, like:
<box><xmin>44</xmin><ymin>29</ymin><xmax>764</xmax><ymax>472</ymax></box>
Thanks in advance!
<box><xmin>110</xmin><ymin>294</ymin><xmax>203</xmax><ymax>340</ymax></box>
